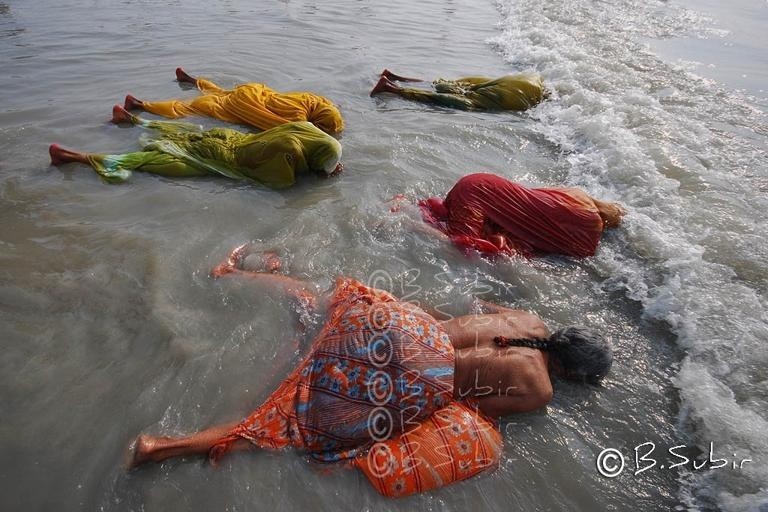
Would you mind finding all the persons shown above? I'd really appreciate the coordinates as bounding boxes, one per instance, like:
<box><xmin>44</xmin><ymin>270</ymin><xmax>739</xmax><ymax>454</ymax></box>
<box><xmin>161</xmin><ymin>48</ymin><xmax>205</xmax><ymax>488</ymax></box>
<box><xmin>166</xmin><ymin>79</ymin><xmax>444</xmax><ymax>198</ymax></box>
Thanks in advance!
<box><xmin>120</xmin><ymin>65</ymin><xmax>348</xmax><ymax>138</ymax></box>
<box><xmin>383</xmin><ymin>171</ymin><xmax>629</xmax><ymax>264</ymax></box>
<box><xmin>122</xmin><ymin>240</ymin><xmax>616</xmax><ymax>499</ymax></box>
<box><xmin>370</xmin><ymin>64</ymin><xmax>555</xmax><ymax>116</ymax></box>
<box><xmin>46</xmin><ymin>102</ymin><xmax>347</xmax><ymax>192</ymax></box>
